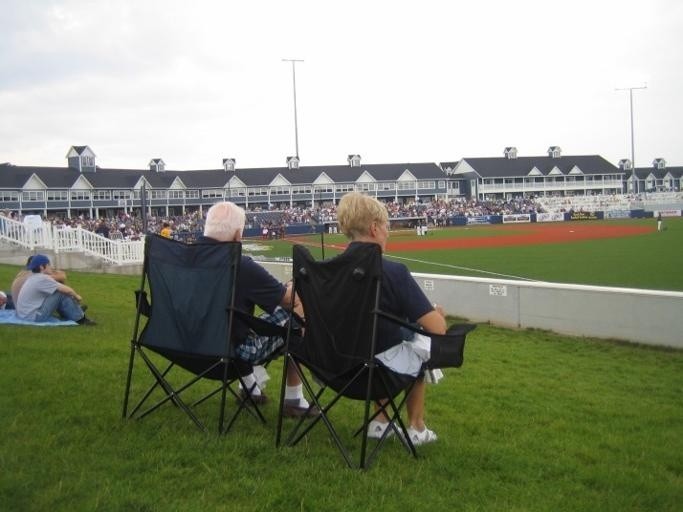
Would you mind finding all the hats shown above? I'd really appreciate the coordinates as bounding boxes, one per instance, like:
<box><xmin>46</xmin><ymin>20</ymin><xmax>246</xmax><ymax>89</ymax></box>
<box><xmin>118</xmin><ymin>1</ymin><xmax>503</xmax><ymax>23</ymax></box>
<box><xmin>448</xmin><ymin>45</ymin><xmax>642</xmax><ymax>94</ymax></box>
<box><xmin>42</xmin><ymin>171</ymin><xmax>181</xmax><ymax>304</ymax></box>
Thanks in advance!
<box><xmin>27</xmin><ymin>255</ymin><xmax>49</xmax><ymax>270</ymax></box>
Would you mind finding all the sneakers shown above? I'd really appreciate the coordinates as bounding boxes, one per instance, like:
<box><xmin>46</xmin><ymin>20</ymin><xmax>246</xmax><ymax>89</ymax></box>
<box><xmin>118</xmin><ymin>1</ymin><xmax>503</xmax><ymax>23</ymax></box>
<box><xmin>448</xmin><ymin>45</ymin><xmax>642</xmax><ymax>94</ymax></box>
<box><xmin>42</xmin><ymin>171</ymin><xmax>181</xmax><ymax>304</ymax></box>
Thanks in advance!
<box><xmin>284</xmin><ymin>404</ymin><xmax>319</xmax><ymax>418</ymax></box>
<box><xmin>236</xmin><ymin>389</ymin><xmax>267</xmax><ymax>404</ymax></box>
<box><xmin>80</xmin><ymin>304</ymin><xmax>88</xmax><ymax>312</ymax></box>
<box><xmin>401</xmin><ymin>426</ymin><xmax>438</xmax><ymax>446</ymax></box>
<box><xmin>78</xmin><ymin>317</ymin><xmax>96</xmax><ymax>326</ymax></box>
<box><xmin>367</xmin><ymin>420</ymin><xmax>396</xmax><ymax>439</ymax></box>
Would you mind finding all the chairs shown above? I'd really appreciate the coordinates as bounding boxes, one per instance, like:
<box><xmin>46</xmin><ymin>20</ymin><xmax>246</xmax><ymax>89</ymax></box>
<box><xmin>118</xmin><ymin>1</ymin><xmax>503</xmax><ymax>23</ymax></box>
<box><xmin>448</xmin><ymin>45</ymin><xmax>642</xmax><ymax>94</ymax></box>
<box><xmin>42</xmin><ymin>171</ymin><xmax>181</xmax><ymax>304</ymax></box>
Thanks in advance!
<box><xmin>128</xmin><ymin>229</ymin><xmax>271</xmax><ymax>439</ymax></box>
<box><xmin>276</xmin><ymin>241</ymin><xmax>476</xmax><ymax>468</ymax></box>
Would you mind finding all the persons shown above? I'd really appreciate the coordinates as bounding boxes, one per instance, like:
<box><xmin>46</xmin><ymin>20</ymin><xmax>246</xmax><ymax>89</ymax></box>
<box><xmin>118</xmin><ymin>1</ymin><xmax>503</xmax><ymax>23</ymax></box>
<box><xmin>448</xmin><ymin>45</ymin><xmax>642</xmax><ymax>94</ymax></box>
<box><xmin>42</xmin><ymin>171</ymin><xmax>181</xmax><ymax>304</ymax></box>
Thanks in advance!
<box><xmin>199</xmin><ymin>197</ymin><xmax>319</xmax><ymax>422</ymax></box>
<box><xmin>656</xmin><ymin>211</ymin><xmax>662</xmax><ymax>232</ymax></box>
<box><xmin>305</xmin><ymin>186</ymin><xmax>449</xmax><ymax>449</ymax></box>
<box><xmin>7</xmin><ymin>192</ymin><xmax>543</xmax><ymax>238</ymax></box>
<box><xmin>8</xmin><ymin>252</ymin><xmax>95</xmax><ymax>326</ymax></box>
